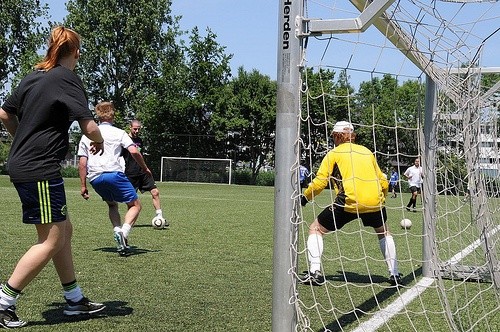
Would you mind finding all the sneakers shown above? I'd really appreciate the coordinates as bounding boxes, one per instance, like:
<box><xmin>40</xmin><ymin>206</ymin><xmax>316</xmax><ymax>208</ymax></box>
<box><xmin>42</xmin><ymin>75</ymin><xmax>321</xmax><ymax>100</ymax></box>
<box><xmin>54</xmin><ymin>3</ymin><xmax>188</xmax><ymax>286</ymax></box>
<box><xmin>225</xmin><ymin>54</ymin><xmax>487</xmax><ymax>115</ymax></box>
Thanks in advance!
<box><xmin>0</xmin><ymin>306</ymin><xmax>28</xmax><ymax>329</ymax></box>
<box><xmin>406</xmin><ymin>207</ymin><xmax>418</xmax><ymax>211</ymax></box>
<box><xmin>166</xmin><ymin>222</ymin><xmax>170</xmax><ymax>227</ymax></box>
<box><xmin>391</xmin><ymin>195</ymin><xmax>398</xmax><ymax>198</ymax></box>
<box><xmin>62</xmin><ymin>296</ymin><xmax>106</xmax><ymax>316</ymax></box>
<box><xmin>299</xmin><ymin>271</ymin><xmax>324</xmax><ymax>286</ymax></box>
<box><xmin>391</xmin><ymin>273</ymin><xmax>405</xmax><ymax>286</ymax></box>
<box><xmin>115</xmin><ymin>232</ymin><xmax>130</xmax><ymax>256</ymax></box>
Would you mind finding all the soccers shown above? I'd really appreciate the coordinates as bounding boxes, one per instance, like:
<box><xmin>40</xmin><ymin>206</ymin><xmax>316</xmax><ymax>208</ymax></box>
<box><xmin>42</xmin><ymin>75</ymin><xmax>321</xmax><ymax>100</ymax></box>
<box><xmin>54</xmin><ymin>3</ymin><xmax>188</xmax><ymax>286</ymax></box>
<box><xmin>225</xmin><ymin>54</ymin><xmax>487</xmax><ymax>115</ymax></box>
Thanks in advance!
<box><xmin>400</xmin><ymin>219</ymin><xmax>412</xmax><ymax>229</ymax></box>
<box><xmin>152</xmin><ymin>216</ymin><xmax>165</xmax><ymax>229</ymax></box>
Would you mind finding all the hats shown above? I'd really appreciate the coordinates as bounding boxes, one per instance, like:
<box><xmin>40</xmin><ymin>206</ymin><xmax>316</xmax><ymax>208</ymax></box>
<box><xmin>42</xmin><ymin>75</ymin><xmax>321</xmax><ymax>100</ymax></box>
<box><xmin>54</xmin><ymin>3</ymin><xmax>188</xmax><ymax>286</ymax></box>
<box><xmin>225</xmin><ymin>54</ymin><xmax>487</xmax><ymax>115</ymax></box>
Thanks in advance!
<box><xmin>330</xmin><ymin>121</ymin><xmax>355</xmax><ymax>134</ymax></box>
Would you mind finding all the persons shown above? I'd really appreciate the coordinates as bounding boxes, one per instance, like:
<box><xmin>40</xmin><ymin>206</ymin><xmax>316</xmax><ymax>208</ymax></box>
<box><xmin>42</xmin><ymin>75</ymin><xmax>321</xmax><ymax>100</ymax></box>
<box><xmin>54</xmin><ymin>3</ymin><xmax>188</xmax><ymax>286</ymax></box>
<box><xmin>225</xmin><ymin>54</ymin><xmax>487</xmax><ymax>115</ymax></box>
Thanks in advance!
<box><xmin>0</xmin><ymin>24</ymin><xmax>108</xmax><ymax>328</ymax></box>
<box><xmin>301</xmin><ymin>120</ymin><xmax>402</xmax><ymax>286</ymax></box>
<box><xmin>76</xmin><ymin>101</ymin><xmax>153</xmax><ymax>256</ymax></box>
<box><xmin>298</xmin><ymin>157</ymin><xmax>423</xmax><ymax>213</ymax></box>
<box><xmin>121</xmin><ymin>119</ymin><xmax>169</xmax><ymax>227</ymax></box>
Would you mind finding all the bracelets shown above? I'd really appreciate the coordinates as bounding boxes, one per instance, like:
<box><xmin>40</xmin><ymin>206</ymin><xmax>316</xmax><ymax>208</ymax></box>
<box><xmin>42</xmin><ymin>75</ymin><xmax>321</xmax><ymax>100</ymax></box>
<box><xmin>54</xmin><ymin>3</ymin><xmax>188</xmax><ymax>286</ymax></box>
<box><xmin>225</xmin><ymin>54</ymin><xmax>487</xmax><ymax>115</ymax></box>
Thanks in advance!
<box><xmin>95</xmin><ymin>139</ymin><xmax>105</xmax><ymax>144</ymax></box>
